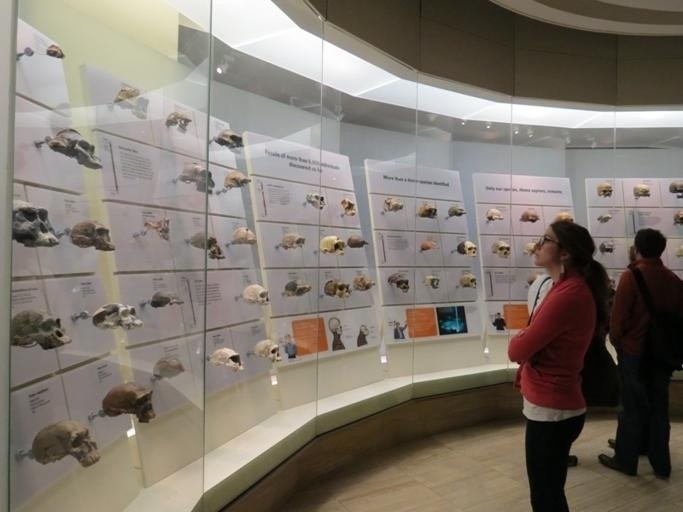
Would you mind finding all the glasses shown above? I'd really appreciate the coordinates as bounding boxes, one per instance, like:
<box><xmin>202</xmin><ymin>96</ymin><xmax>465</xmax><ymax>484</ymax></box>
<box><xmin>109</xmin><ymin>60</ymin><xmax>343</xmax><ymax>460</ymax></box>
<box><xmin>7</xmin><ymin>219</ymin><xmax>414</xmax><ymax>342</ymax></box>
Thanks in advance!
<box><xmin>539</xmin><ymin>234</ymin><xmax>557</xmax><ymax>247</ymax></box>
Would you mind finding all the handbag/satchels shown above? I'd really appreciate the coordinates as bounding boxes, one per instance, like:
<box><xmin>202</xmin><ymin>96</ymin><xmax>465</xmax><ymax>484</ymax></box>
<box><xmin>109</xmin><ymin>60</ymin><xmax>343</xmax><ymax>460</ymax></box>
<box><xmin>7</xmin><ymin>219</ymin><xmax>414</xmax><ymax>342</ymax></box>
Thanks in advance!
<box><xmin>582</xmin><ymin>339</ymin><xmax>621</xmax><ymax>406</ymax></box>
<box><xmin>646</xmin><ymin>311</ymin><xmax>683</xmax><ymax>370</ymax></box>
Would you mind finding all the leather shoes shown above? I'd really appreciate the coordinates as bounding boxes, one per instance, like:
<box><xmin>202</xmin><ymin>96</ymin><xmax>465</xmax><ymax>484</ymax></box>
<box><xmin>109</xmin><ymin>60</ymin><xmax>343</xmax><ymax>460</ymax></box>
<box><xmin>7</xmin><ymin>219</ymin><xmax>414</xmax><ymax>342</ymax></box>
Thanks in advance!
<box><xmin>599</xmin><ymin>453</ymin><xmax>637</xmax><ymax>475</ymax></box>
<box><xmin>608</xmin><ymin>439</ymin><xmax>617</xmax><ymax>447</ymax></box>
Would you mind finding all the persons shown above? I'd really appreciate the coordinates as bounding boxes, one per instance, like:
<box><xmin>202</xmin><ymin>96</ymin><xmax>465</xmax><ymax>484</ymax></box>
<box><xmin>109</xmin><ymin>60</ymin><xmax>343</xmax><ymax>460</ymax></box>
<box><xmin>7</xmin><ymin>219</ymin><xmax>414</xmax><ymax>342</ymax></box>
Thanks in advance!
<box><xmin>492</xmin><ymin>312</ymin><xmax>505</xmax><ymax>331</ymax></box>
<box><xmin>285</xmin><ymin>335</ymin><xmax>297</xmax><ymax>359</ymax></box>
<box><xmin>395</xmin><ymin>322</ymin><xmax>407</xmax><ymax>339</ymax></box>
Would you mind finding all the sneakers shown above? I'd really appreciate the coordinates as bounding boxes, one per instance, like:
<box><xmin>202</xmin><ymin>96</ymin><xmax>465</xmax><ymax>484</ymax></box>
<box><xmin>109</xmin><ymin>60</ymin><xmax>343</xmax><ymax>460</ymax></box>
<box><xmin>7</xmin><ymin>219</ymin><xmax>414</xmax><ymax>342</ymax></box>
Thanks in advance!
<box><xmin>568</xmin><ymin>455</ymin><xmax>578</xmax><ymax>466</ymax></box>
